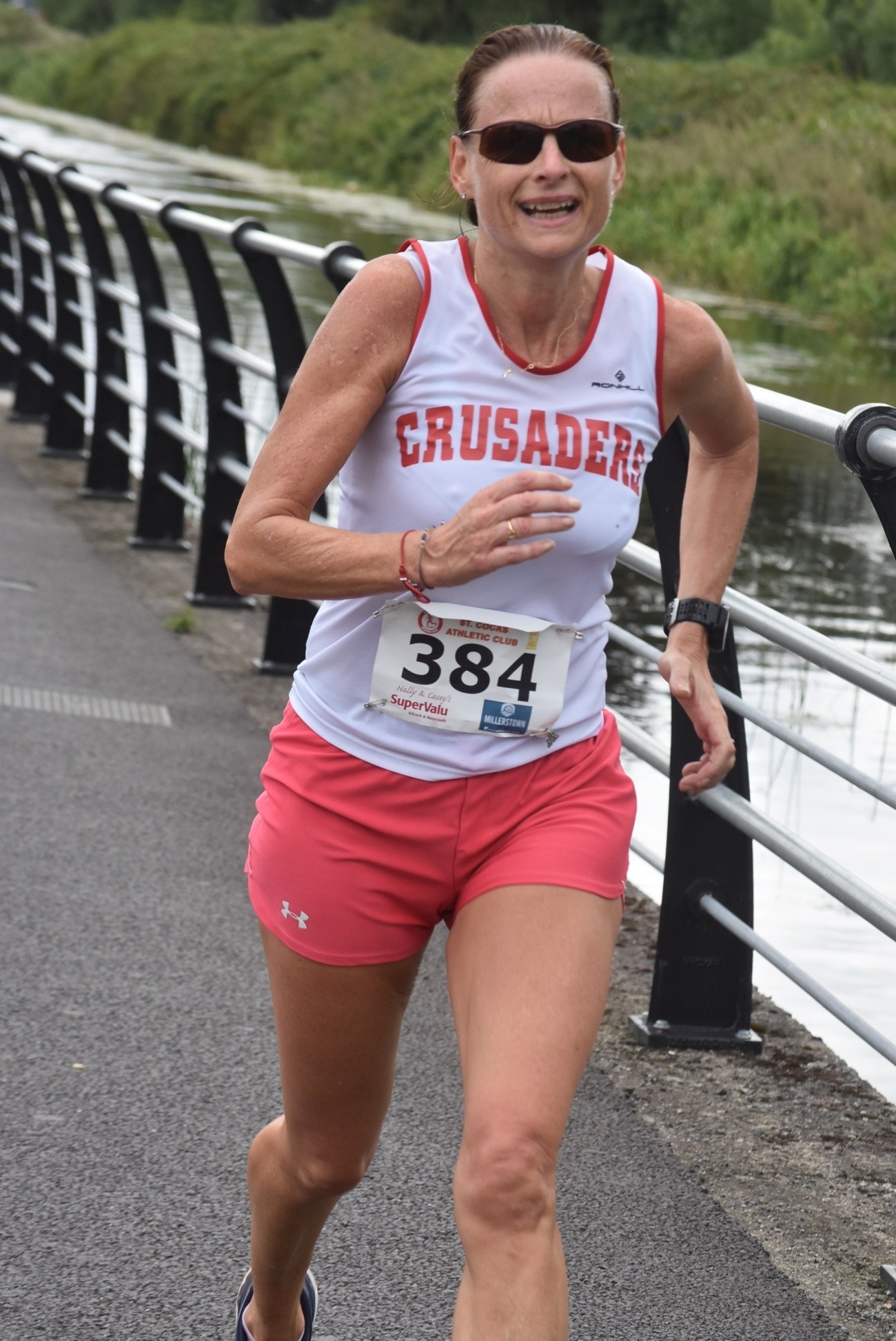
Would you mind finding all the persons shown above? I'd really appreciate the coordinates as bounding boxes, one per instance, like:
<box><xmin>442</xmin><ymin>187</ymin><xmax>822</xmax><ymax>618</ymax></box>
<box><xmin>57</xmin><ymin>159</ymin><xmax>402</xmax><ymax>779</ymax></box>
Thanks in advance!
<box><xmin>223</xmin><ymin>23</ymin><xmax>759</xmax><ymax>1341</ymax></box>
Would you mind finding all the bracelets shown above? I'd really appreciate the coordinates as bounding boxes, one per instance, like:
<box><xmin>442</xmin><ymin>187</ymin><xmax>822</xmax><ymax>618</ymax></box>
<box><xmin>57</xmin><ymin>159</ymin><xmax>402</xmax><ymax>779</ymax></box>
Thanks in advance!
<box><xmin>397</xmin><ymin>520</ymin><xmax>447</xmax><ymax>603</ymax></box>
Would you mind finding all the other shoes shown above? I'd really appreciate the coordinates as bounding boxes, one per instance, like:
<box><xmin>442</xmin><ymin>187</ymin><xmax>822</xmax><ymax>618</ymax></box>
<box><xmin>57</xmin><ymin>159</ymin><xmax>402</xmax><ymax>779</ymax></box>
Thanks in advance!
<box><xmin>235</xmin><ymin>1267</ymin><xmax>318</xmax><ymax>1341</ymax></box>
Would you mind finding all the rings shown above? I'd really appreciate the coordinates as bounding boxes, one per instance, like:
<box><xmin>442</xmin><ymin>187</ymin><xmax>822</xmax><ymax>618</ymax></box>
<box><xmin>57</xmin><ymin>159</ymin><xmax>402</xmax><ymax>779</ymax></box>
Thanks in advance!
<box><xmin>506</xmin><ymin>519</ymin><xmax>517</xmax><ymax>540</ymax></box>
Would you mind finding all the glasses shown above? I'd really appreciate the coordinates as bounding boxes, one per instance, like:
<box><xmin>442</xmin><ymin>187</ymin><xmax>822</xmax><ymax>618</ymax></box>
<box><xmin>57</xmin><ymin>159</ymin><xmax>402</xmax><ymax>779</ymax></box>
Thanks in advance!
<box><xmin>459</xmin><ymin>118</ymin><xmax>624</xmax><ymax>164</ymax></box>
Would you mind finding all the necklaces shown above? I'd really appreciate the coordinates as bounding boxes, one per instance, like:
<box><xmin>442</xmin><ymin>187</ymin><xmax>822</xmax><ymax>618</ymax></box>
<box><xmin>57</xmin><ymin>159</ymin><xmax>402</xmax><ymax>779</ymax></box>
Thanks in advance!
<box><xmin>471</xmin><ymin>249</ymin><xmax>588</xmax><ymax>379</ymax></box>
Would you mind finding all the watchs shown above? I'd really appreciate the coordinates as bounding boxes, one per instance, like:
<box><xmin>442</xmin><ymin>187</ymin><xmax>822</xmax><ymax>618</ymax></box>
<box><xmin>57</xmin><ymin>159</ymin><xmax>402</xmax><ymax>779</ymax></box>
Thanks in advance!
<box><xmin>663</xmin><ymin>597</ymin><xmax>732</xmax><ymax>655</ymax></box>
